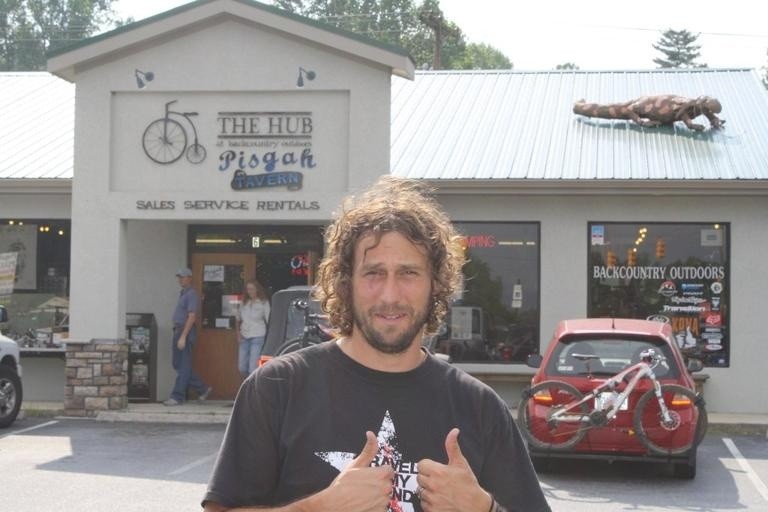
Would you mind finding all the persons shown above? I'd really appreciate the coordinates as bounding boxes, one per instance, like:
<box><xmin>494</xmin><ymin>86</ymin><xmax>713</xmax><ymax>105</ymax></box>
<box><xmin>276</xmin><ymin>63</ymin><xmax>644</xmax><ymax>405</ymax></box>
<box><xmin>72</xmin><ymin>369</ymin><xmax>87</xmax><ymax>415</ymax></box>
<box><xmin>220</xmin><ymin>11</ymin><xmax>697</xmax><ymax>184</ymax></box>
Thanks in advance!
<box><xmin>200</xmin><ymin>174</ymin><xmax>554</xmax><ymax>512</ymax></box>
<box><xmin>161</xmin><ymin>266</ymin><xmax>212</xmax><ymax>406</ymax></box>
<box><xmin>234</xmin><ymin>277</ymin><xmax>272</xmax><ymax>382</ymax></box>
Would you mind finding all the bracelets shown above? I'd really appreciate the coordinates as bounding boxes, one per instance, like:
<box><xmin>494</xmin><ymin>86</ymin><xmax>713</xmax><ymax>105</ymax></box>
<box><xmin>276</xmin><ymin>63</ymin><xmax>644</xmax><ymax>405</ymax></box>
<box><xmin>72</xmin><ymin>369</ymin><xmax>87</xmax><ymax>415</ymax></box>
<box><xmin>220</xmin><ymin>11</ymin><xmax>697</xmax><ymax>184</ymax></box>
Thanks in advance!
<box><xmin>486</xmin><ymin>493</ymin><xmax>505</xmax><ymax>512</ymax></box>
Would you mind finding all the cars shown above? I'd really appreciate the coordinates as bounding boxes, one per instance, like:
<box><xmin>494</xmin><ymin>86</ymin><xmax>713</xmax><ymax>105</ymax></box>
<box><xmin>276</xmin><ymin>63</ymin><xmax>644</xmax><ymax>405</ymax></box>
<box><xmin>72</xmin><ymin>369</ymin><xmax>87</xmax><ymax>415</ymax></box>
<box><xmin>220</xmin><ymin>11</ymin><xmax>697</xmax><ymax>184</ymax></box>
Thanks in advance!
<box><xmin>256</xmin><ymin>283</ymin><xmax>335</xmax><ymax>366</ymax></box>
<box><xmin>0</xmin><ymin>305</ymin><xmax>24</xmax><ymax>429</ymax></box>
<box><xmin>479</xmin><ymin>324</ymin><xmax>538</xmax><ymax>363</ymax></box>
<box><xmin>525</xmin><ymin>317</ymin><xmax>704</xmax><ymax>478</ymax></box>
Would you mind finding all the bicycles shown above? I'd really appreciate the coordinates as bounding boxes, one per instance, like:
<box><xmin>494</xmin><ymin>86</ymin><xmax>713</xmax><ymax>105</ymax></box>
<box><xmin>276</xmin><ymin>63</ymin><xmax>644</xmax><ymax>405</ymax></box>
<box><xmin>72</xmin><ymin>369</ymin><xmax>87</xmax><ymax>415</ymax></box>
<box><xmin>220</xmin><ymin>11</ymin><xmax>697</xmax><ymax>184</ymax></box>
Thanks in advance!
<box><xmin>517</xmin><ymin>348</ymin><xmax>708</xmax><ymax>456</ymax></box>
<box><xmin>273</xmin><ymin>299</ymin><xmax>337</xmax><ymax>358</ymax></box>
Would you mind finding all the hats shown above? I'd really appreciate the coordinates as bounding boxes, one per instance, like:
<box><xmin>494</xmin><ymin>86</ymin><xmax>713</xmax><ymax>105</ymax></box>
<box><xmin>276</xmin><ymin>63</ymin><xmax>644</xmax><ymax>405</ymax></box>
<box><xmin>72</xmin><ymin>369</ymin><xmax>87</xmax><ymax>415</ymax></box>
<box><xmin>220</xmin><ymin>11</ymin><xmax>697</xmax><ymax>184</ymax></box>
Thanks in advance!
<box><xmin>175</xmin><ymin>267</ymin><xmax>193</xmax><ymax>279</ymax></box>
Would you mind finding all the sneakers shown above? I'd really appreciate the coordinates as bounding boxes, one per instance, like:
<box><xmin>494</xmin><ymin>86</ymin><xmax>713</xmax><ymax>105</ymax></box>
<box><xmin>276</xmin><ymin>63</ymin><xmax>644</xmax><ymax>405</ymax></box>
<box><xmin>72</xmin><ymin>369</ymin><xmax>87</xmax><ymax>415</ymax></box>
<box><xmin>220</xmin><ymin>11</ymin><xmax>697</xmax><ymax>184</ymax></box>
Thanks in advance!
<box><xmin>198</xmin><ymin>387</ymin><xmax>212</xmax><ymax>403</ymax></box>
<box><xmin>163</xmin><ymin>397</ymin><xmax>185</xmax><ymax>407</ymax></box>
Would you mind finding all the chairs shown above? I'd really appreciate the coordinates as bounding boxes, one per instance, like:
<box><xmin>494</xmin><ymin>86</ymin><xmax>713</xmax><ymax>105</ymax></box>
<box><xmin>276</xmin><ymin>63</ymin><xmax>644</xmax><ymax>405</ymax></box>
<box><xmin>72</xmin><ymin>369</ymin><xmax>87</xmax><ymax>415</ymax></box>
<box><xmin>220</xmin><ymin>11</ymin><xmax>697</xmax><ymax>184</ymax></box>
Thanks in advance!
<box><xmin>564</xmin><ymin>341</ymin><xmax>667</xmax><ymax>378</ymax></box>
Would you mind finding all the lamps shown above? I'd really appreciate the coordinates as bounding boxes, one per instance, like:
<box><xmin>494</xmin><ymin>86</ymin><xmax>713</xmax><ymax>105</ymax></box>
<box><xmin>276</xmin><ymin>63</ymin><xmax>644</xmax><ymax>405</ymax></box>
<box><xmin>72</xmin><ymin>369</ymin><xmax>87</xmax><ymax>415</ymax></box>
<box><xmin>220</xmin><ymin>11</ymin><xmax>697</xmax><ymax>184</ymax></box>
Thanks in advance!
<box><xmin>295</xmin><ymin>67</ymin><xmax>316</xmax><ymax>88</ymax></box>
<box><xmin>135</xmin><ymin>69</ymin><xmax>155</xmax><ymax>91</ymax></box>
